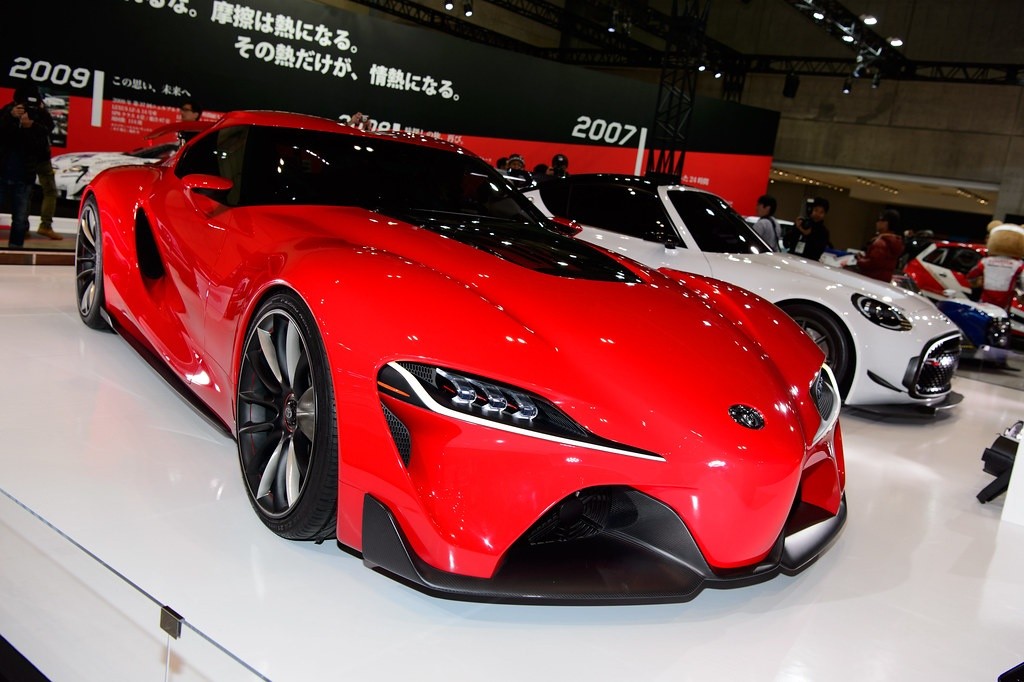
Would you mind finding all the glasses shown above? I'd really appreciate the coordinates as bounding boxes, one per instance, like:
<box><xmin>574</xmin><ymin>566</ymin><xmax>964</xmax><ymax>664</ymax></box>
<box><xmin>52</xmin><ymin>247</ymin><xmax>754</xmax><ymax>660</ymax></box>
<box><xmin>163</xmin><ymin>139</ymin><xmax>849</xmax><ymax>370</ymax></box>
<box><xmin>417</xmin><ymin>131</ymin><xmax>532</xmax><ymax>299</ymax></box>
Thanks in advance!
<box><xmin>183</xmin><ymin>108</ymin><xmax>192</xmax><ymax>112</ymax></box>
<box><xmin>876</xmin><ymin>218</ymin><xmax>885</xmax><ymax>222</ymax></box>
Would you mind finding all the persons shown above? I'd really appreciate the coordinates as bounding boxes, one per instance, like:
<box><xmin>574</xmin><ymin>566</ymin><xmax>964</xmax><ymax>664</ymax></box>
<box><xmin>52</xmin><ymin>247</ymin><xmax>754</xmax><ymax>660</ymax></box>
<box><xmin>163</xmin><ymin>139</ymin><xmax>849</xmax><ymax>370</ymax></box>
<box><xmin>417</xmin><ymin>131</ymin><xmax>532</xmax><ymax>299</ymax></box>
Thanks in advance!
<box><xmin>966</xmin><ymin>220</ymin><xmax>1024</xmax><ymax>309</ymax></box>
<box><xmin>181</xmin><ymin>99</ymin><xmax>203</xmax><ymax>121</ymax></box>
<box><xmin>0</xmin><ymin>85</ymin><xmax>56</xmax><ymax>248</ymax></box>
<box><xmin>783</xmin><ymin>198</ymin><xmax>830</xmax><ymax>261</ymax></box>
<box><xmin>497</xmin><ymin>153</ymin><xmax>526</xmax><ymax>170</ymax></box>
<box><xmin>752</xmin><ymin>195</ymin><xmax>782</xmax><ymax>253</ymax></box>
<box><xmin>855</xmin><ymin>210</ymin><xmax>906</xmax><ymax>283</ymax></box>
<box><xmin>546</xmin><ymin>154</ymin><xmax>572</xmax><ymax>179</ymax></box>
<box><xmin>26</xmin><ymin>137</ymin><xmax>63</xmax><ymax>240</ymax></box>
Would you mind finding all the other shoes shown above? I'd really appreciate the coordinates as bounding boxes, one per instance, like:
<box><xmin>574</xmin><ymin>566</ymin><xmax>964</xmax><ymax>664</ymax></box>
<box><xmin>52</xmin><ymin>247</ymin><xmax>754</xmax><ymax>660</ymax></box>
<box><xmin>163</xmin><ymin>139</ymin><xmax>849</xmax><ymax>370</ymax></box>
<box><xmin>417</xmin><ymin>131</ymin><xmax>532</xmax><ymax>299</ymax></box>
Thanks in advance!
<box><xmin>37</xmin><ymin>225</ymin><xmax>62</xmax><ymax>240</ymax></box>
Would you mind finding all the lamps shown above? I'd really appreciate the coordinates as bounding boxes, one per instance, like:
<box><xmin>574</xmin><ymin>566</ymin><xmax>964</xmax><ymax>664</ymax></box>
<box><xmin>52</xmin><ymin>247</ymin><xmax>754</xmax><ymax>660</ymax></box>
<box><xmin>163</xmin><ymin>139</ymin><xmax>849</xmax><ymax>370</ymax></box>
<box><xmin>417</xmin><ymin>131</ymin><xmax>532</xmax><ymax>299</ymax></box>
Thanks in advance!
<box><xmin>842</xmin><ymin>24</ymin><xmax>855</xmax><ymax>42</ymax></box>
<box><xmin>853</xmin><ymin>64</ymin><xmax>865</xmax><ymax>78</ymax></box>
<box><xmin>607</xmin><ymin>18</ymin><xmax>617</xmax><ymax>32</ymax></box>
<box><xmin>868</xmin><ymin>40</ymin><xmax>882</xmax><ymax>55</ymax></box>
<box><xmin>464</xmin><ymin>3</ymin><xmax>473</xmax><ymax>17</ymax></box>
<box><xmin>444</xmin><ymin>0</ymin><xmax>454</xmax><ymax>10</ymax></box>
<box><xmin>871</xmin><ymin>72</ymin><xmax>882</xmax><ymax>89</ymax></box>
<box><xmin>861</xmin><ymin>10</ymin><xmax>878</xmax><ymax>26</ymax></box>
<box><xmin>843</xmin><ymin>76</ymin><xmax>851</xmax><ymax>94</ymax></box>
<box><xmin>890</xmin><ymin>34</ymin><xmax>905</xmax><ymax>47</ymax></box>
<box><xmin>697</xmin><ymin>60</ymin><xmax>705</xmax><ymax>72</ymax></box>
<box><xmin>712</xmin><ymin>68</ymin><xmax>722</xmax><ymax>79</ymax></box>
<box><xmin>856</xmin><ymin>48</ymin><xmax>864</xmax><ymax>62</ymax></box>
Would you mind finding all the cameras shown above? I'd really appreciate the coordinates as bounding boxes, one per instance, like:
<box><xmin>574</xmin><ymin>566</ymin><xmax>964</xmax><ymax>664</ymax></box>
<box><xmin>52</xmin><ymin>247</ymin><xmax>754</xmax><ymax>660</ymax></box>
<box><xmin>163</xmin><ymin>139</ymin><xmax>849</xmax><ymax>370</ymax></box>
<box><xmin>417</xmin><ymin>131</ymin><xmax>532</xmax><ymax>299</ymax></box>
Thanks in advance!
<box><xmin>23</xmin><ymin>96</ymin><xmax>41</xmax><ymax>122</ymax></box>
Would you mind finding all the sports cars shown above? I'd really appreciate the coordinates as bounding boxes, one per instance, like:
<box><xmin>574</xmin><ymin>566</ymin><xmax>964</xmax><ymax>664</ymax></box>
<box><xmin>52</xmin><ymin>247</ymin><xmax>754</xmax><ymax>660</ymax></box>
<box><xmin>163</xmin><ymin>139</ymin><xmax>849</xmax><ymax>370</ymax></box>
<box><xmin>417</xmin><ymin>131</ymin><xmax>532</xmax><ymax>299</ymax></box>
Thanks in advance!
<box><xmin>498</xmin><ymin>170</ymin><xmax>963</xmax><ymax>423</ymax></box>
<box><xmin>74</xmin><ymin>109</ymin><xmax>849</xmax><ymax>605</ymax></box>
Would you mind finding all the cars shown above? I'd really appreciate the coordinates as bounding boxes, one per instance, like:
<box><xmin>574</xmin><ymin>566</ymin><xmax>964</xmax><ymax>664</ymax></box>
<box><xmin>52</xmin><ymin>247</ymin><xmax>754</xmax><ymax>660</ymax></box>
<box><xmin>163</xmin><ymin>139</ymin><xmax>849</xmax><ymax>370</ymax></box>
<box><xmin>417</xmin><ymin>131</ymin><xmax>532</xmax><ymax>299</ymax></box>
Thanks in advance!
<box><xmin>904</xmin><ymin>236</ymin><xmax>1024</xmax><ymax>350</ymax></box>
<box><xmin>35</xmin><ymin>121</ymin><xmax>225</xmax><ymax>217</ymax></box>
<box><xmin>742</xmin><ymin>215</ymin><xmax>1009</xmax><ymax>359</ymax></box>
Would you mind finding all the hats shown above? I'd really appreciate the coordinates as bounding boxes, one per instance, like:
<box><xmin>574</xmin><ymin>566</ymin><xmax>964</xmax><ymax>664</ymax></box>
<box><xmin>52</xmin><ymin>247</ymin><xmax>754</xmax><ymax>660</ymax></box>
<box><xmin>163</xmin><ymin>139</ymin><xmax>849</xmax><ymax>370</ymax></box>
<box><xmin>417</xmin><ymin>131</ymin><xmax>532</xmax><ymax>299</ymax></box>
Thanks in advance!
<box><xmin>507</xmin><ymin>154</ymin><xmax>524</xmax><ymax>167</ymax></box>
<box><xmin>815</xmin><ymin>197</ymin><xmax>829</xmax><ymax>213</ymax></box>
<box><xmin>553</xmin><ymin>154</ymin><xmax>568</xmax><ymax>167</ymax></box>
